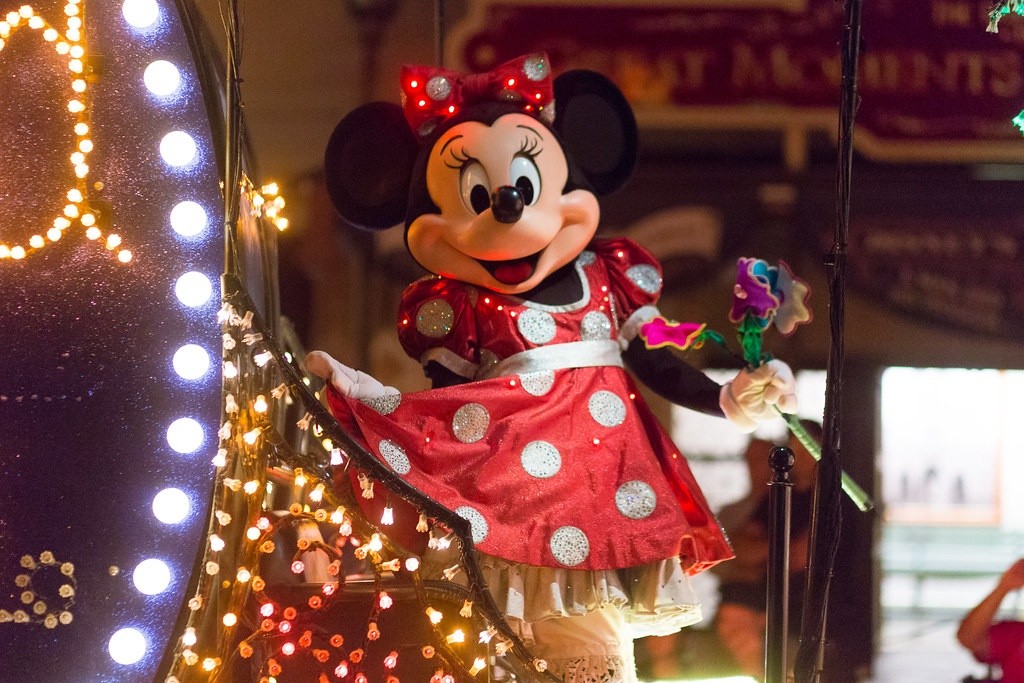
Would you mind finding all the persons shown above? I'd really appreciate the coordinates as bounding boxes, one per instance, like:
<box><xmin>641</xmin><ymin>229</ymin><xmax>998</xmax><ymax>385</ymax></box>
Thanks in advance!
<box><xmin>957</xmin><ymin>558</ymin><xmax>1024</xmax><ymax>683</ymax></box>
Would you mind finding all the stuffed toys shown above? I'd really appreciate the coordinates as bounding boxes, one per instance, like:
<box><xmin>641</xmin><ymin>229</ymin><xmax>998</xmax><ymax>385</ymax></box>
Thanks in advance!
<box><xmin>304</xmin><ymin>51</ymin><xmax>800</xmax><ymax>683</ymax></box>
